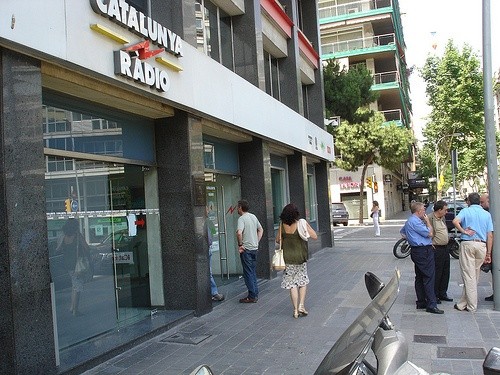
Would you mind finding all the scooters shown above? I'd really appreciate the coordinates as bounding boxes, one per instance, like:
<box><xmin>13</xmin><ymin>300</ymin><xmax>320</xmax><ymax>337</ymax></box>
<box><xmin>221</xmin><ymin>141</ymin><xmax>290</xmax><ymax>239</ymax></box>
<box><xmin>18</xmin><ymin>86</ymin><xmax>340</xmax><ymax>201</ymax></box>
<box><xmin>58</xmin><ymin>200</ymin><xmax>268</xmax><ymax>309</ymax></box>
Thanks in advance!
<box><xmin>393</xmin><ymin>235</ymin><xmax>462</xmax><ymax>260</ymax></box>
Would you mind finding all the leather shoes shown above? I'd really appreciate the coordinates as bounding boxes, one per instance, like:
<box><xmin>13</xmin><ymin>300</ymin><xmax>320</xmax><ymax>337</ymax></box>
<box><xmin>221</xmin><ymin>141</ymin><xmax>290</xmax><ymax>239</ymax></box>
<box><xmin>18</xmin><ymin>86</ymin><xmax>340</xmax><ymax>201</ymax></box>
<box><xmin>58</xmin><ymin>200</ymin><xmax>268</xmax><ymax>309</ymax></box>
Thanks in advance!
<box><xmin>441</xmin><ymin>297</ymin><xmax>453</xmax><ymax>301</ymax></box>
<box><xmin>436</xmin><ymin>298</ymin><xmax>441</xmax><ymax>304</ymax></box>
<box><xmin>485</xmin><ymin>294</ymin><xmax>493</xmax><ymax>301</ymax></box>
<box><xmin>240</xmin><ymin>297</ymin><xmax>257</xmax><ymax>303</ymax></box>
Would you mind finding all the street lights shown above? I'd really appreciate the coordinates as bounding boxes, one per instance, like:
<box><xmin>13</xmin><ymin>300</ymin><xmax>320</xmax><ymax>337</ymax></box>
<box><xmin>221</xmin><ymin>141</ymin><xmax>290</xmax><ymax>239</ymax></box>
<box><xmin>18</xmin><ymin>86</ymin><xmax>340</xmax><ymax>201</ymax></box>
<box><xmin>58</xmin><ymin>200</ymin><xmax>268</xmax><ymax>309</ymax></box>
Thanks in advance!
<box><xmin>62</xmin><ymin>117</ymin><xmax>82</xmax><ymax>233</ymax></box>
<box><xmin>434</xmin><ymin>133</ymin><xmax>464</xmax><ymax>201</ymax></box>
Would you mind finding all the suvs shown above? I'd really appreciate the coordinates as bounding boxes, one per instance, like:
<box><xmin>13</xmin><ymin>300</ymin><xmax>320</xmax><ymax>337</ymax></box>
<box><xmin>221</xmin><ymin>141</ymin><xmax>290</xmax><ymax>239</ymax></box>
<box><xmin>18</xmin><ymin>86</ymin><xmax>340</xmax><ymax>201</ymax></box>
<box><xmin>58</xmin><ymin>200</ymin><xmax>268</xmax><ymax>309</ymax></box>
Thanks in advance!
<box><xmin>330</xmin><ymin>203</ymin><xmax>349</xmax><ymax>226</ymax></box>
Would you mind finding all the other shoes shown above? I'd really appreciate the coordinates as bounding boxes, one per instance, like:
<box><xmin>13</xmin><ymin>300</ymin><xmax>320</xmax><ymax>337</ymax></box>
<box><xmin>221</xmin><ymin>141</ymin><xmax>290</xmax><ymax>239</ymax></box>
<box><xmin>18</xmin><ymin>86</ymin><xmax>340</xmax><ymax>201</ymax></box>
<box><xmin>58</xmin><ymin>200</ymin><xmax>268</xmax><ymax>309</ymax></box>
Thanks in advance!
<box><xmin>454</xmin><ymin>304</ymin><xmax>459</xmax><ymax>310</ymax></box>
<box><xmin>426</xmin><ymin>307</ymin><xmax>445</xmax><ymax>314</ymax></box>
<box><xmin>293</xmin><ymin>310</ymin><xmax>299</xmax><ymax>319</ymax></box>
<box><xmin>212</xmin><ymin>293</ymin><xmax>224</xmax><ymax>301</ymax></box>
<box><xmin>298</xmin><ymin>304</ymin><xmax>309</xmax><ymax>314</ymax></box>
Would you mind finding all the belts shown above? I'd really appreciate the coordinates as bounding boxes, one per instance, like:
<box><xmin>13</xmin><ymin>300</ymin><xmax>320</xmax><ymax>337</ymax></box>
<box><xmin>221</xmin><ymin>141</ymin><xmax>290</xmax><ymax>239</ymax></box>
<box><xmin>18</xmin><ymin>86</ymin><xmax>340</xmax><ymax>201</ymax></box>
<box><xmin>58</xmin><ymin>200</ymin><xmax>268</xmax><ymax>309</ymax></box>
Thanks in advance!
<box><xmin>461</xmin><ymin>239</ymin><xmax>486</xmax><ymax>243</ymax></box>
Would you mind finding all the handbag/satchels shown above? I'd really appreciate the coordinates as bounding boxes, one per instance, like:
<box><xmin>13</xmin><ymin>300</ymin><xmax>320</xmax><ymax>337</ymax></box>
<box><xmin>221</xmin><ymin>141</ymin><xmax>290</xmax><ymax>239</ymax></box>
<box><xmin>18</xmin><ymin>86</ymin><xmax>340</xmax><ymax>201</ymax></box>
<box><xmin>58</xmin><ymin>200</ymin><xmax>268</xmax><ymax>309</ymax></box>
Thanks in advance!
<box><xmin>272</xmin><ymin>249</ymin><xmax>285</xmax><ymax>270</ymax></box>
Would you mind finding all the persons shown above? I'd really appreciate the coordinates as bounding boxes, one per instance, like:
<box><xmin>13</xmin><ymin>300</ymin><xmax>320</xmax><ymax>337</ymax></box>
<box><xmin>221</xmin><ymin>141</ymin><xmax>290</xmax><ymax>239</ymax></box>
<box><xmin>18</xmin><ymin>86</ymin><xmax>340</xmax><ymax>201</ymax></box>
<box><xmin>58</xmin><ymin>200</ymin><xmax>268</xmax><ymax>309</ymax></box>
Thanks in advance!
<box><xmin>276</xmin><ymin>204</ymin><xmax>317</xmax><ymax>318</ymax></box>
<box><xmin>400</xmin><ymin>202</ymin><xmax>444</xmax><ymax>314</ymax></box>
<box><xmin>206</xmin><ymin>206</ymin><xmax>224</xmax><ymax>302</ymax></box>
<box><xmin>424</xmin><ymin>200</ymin><xmax>453</xmax><ymax>304</ymax></box>
<box><xmin>237</xmin><ymin>199</ymin><xmax>263</xmax><ymax>303</ymax></box>
<box><xmin>452</xmin><ymin>193</ymin><xmax>494</xmax><ymax>312</ymax></box>
<box><xmin>57</xmin><ymin>218</ymin><xmax>90</xmax><ymax>317</ymax></box>
<box><xmin>371</xmin><ymin>201</ymin><xmax>380</xmax><ymax>236</ymax></box>
<box><xmin>479</xmin><ymin>194</ymin><xmax>494</xmax><ymax>301</ymax></box>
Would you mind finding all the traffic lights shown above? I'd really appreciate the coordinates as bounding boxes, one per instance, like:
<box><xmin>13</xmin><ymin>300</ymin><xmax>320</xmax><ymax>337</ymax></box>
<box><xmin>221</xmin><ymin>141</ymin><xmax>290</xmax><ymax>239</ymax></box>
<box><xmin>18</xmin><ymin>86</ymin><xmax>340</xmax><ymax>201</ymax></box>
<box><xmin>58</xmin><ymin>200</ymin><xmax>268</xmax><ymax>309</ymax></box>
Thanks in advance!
<box><xmin>65</xmin><ymin>199</ymin><xmax>71</xmax><ymax>213</ymax></box>
<box><xmin>135</xmin><ymin>214</ymin><xmax>147</xmax><ymax>230</ymax></box>
<box><xmin>374</xmin><ymin>182</ymin><xmax>377</xmax><ymax>190</ymax></box>
<box><xmin>366</xmin><ymin>177</ymin><xmax>372</xmax><ymax>188</ymax></box>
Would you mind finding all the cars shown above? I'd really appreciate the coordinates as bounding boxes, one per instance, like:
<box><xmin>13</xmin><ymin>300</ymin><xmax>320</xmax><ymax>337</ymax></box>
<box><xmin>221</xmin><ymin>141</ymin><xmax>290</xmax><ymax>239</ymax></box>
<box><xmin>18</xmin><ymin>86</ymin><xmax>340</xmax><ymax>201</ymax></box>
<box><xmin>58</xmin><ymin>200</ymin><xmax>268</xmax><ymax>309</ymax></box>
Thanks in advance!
<box><xmin>47</xmin><ymin>237</ymin><xmax>73</xmax><ymax>287</ymax></box>
<box><xmin>444</xmin><ymin>201</ymin><xmax>468</xmax><ymax>232</ymax></box>
<box><xmin>94</xmin><ymin>229</ymin><xmax>140</xmax><ymax>275</ymax></box>
